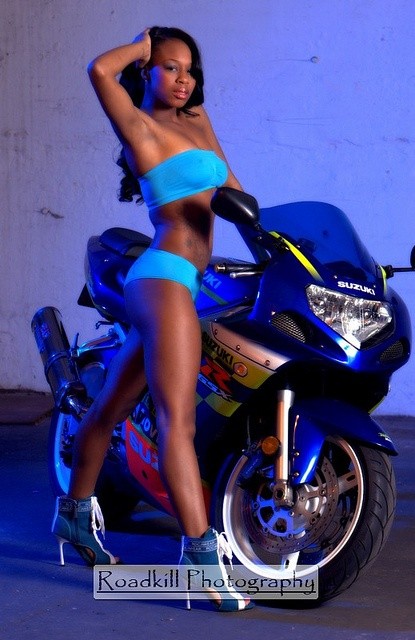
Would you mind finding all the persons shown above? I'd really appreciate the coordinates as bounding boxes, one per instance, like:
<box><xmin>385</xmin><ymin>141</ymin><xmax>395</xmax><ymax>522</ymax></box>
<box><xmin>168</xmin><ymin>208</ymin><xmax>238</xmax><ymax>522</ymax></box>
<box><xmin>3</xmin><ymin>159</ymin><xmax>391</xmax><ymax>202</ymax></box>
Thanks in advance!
<box><xmin>52</xmin><ymin>26</ymin><xmax>271</xmax><ymax>611</ymax></box>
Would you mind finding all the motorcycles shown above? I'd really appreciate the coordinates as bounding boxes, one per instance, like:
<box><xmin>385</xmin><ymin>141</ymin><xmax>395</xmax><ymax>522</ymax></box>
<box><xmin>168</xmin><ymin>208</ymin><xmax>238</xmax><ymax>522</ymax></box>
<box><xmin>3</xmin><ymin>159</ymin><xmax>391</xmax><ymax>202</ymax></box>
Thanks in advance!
<box><xmin>29</xmin><ymin>185</ymin><xmax>415</xmax><ymax>612</ymax></box>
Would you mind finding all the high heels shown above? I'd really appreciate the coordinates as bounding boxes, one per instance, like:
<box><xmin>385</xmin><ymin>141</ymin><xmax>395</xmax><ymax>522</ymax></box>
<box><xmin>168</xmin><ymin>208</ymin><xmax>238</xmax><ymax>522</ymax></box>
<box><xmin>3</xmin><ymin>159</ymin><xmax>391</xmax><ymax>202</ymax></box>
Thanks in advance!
<box><xmin>177</xmin><ymin>526</ymin><xmax>257</xmax><ymax>613</ymax></box>
<box><xmin>51</xmin><ymin>493</ymin><xmax>122</xmax><ymax>568</ymax></box>
